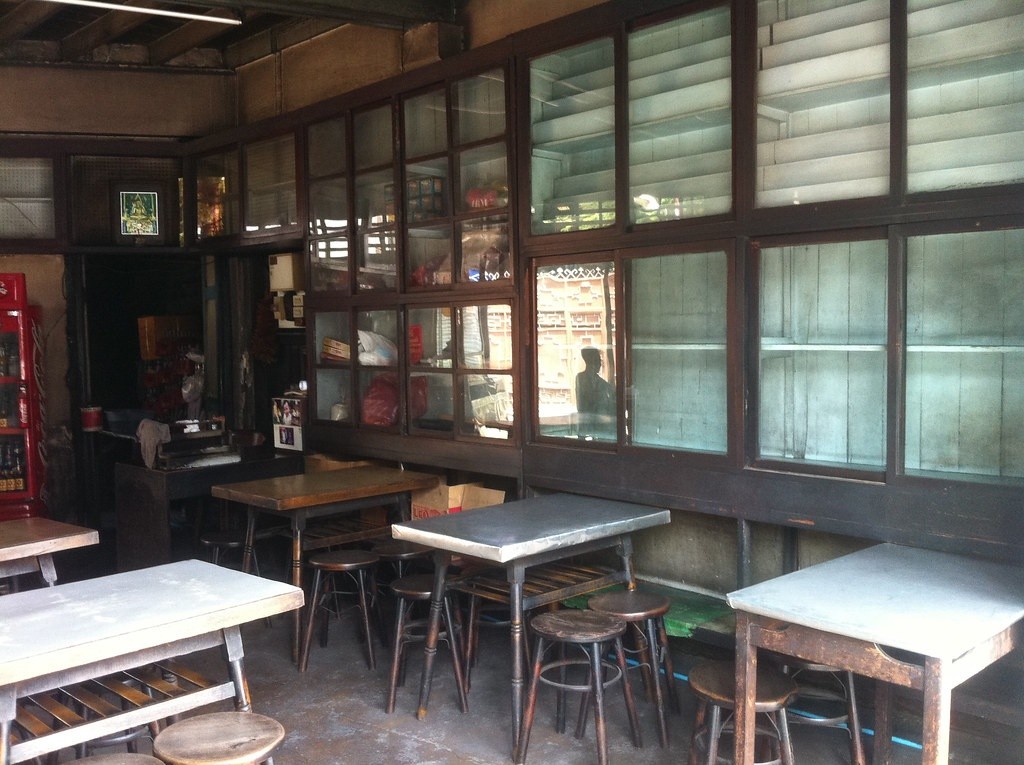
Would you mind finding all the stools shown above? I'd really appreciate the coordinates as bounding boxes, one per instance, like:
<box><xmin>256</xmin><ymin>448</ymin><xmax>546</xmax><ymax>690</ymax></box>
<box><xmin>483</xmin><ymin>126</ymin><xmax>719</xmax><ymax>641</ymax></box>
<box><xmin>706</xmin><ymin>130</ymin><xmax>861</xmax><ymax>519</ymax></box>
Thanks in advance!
<box><xmin>575</xmin><ymin>589</ymin><xmax>677</xmax><ymax>749</ymax></box>
<box><xmin>54</xmin><ymin>678</ymin><xmax>162</xmax><ymax>760</ymax></box>
<box><xmin>152</xmin><ymin>711</ymin><xmax>286</xmax><ymax>765</ymax></box>
<box><xmin>516</xmin><ymin>609</ymin><xmax>643</xmax><ymax>765</ymax></box>
<box><xmin>200</xmin><ymin>530</ymin><xmax>273</xmax><ymax>628</ymax></box>
<box><xmin>297</xmin><ymin>540</ymin><xmax>539</xmax><ymax>714</ymax></box>
<box><xmin>688</xmin><ymin>657</ymin><xmax>796</xmax><ymax>765</ymax></box>
<box><xmin>763</xmin><ymin>652</ymin><xmax>867</xmax><ymax>765</ymax></box>
<box><xmin>57</xmin><ymin>753</ymin><xmax>166</xmax><ymax>765</ymax></box>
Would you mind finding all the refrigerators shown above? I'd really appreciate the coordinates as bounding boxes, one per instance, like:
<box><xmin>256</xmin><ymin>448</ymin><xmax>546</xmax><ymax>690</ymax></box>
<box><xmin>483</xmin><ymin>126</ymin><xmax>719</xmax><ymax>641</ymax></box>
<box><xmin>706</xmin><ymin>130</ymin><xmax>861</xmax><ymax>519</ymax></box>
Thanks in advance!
<box><xmin>0</xmin><ymin>273</ymin><xmax>48</xmax><ymax>520</ymax></box>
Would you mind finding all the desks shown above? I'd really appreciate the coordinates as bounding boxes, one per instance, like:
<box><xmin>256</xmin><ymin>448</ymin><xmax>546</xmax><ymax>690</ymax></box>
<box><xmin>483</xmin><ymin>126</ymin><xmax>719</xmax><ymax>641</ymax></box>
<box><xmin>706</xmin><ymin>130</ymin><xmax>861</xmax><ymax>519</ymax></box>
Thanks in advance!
<box><xmin>725</xmin><ymin>543</ymin><xmax>1024</xmax><ymax>765</ymax></box>
<box><xmin>211</xmin><ymin>466</ymin><xmax>438</xmax><ymax>666</ymax></box>
<box><xmin>0</xmin><ymin>558</ymin><xmax>305</xmax><ymax>765</ymax></box>
<box><xmin>391</xmin><ymin>492</ymin><xmax>670</xmax><ymax>763</ymax></box>
<box><xmin>0</xmin><ymin>516</ymin><xmax>99</xmax><ymax>596</ymax></box>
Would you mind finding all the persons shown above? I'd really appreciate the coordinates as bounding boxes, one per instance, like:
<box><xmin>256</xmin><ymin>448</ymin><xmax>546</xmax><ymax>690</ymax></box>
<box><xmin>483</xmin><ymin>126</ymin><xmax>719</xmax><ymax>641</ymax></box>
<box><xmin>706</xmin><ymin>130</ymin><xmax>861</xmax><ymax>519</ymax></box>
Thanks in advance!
<box><xmin>282</xmin><ymin>401</ymin><xmax>292</xmax><ymax>426</ymax></box>
<box><xmin>576</xmin><ymin>347</ymin><xmax>618</xmax><ymax>415</ymax></box>
<box><xmin>129</xmin><ymin>197</ymin><xmax>149</xmax><ymax>220</ymax></box>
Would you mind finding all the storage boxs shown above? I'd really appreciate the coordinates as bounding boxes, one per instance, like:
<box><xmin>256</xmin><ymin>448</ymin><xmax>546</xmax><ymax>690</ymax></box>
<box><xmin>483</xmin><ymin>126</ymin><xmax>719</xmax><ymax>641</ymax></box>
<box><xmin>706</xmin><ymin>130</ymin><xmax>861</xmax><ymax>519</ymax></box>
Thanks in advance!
<box><xmin>409</xmin><ymin>480</ymin><xmax>506</xmax><ymax>562</ymax></box>
<box><xmin>303</xmin><ymin>454</ymin><xmax>368</xmax><ymax>473</ymax></box>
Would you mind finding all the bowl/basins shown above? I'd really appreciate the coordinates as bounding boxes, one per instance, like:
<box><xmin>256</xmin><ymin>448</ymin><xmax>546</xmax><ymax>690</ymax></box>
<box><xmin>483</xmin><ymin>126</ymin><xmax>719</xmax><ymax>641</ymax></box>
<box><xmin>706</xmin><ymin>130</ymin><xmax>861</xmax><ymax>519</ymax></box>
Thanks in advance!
<box><xmin>104</xmin><ymin>409</ymin><xmax>155</xmax><ymax>434</ymax></box>
<box><xmin>237</xmin><ymin>432</ymin><xmax>267</xmax><ymax>459</ymax></box>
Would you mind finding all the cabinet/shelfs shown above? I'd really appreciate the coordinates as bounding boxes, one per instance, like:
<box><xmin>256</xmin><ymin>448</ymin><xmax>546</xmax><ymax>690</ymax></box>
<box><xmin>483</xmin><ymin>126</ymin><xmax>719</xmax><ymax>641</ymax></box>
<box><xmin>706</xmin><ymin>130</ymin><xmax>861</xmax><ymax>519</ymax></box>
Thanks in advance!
<box><xmin>115</xmin><ymin>449</ymin><xmax>302</xmax><ymax>573</ymax></box>
